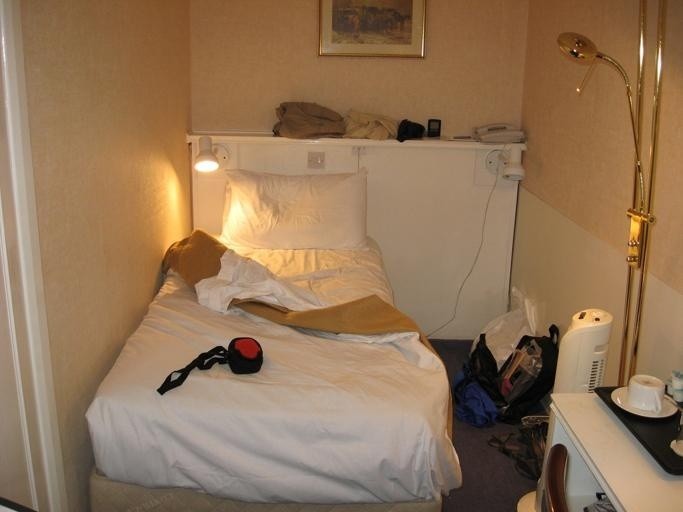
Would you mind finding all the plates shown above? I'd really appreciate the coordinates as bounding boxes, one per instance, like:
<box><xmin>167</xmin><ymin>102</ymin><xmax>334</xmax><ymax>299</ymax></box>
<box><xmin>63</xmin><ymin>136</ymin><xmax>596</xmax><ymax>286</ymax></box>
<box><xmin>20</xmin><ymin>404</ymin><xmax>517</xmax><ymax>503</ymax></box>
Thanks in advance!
<box><xmin>610</xmin><ymin>386</ymin><xmax>677</xmax><ymax>420</ymax></box>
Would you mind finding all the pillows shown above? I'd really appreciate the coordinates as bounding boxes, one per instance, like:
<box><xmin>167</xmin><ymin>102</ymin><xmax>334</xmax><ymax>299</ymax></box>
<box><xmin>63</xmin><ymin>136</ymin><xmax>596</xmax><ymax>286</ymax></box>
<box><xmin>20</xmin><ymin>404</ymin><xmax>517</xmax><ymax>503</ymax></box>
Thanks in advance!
<box><xmin>222</xmin><ymin>168</ymin><xmax>374</xmax><ymax>249</ymax></box>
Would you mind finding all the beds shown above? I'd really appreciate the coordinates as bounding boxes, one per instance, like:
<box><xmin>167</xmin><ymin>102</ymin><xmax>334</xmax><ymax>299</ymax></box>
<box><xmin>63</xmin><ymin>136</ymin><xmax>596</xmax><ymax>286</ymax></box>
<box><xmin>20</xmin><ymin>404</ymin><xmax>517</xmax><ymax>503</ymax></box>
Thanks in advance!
<box><xmin>83</xmin><ymin>258</ymin><xmax>462</xmax><ymax>511</ymax></box>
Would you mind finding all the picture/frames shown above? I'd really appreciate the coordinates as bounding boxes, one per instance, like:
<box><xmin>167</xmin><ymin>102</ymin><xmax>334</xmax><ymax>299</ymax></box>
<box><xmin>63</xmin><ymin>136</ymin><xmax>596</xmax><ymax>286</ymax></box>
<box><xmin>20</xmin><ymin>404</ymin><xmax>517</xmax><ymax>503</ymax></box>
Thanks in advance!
<box><xmin>315</xmin><ymin>0</ymin><xmax>429</xmax><ymax>59</ymax></box>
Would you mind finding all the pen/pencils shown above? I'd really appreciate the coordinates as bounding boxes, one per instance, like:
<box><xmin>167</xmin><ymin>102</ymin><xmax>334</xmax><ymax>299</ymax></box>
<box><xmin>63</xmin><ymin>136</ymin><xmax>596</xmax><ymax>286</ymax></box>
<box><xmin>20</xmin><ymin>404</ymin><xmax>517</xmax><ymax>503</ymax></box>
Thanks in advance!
<box><xmin>454</xmin><ymin>136</ymin><xmax>471</xmax><ymax>139</ymax></box>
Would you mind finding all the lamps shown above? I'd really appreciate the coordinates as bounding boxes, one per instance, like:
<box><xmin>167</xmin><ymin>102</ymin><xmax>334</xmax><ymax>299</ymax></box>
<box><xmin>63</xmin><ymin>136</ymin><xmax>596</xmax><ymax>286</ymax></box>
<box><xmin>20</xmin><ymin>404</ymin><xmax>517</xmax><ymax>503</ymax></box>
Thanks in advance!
<box><xmin>557</xmin><ymin>1</ymin><xmax>673</xmax><ymax>385</ymax></box>
<box><xmin>192</xmin><ymin>133</ymin><xmax>220</xmax><ymax>173</ymax></box>
<box><xmin>484</xmin><ymin>143</ymin><xmax>527</xmax><ymax>186</ymax></box>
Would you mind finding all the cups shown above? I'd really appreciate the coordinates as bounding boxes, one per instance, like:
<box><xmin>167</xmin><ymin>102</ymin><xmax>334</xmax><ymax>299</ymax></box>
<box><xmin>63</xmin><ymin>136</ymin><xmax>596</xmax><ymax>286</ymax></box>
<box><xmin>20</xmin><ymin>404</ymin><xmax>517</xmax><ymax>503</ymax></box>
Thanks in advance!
<box><xmin>628</xmin><ymin>374</ymin><xmax>667</xmax><ymax>414</ymax></box>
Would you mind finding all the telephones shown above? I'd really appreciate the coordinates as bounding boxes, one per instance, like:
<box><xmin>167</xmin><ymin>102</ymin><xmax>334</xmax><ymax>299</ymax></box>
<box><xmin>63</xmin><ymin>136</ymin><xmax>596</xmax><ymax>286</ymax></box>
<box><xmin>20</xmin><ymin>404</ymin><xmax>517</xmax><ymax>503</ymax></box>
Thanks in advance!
<box><xmin>475</xmin><ymin>123</ymin><xmax>524</xmax><ymax>143</ymax></box>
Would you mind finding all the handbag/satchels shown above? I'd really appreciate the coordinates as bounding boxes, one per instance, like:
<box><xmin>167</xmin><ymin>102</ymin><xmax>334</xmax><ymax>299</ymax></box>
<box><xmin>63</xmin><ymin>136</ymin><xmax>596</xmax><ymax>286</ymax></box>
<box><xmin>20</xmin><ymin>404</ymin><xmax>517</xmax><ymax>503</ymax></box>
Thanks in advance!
<box><xmin>451</xmin><ymin>325</ymin><xmax>560</xmax><ymax>425</ymax></box>
<box><xmin>224</xmin><ymin>338</ymin><xmax>263</xmax><ymax>373</ymax></box>
<box><xmin>487</xmin><ymin>421</ymin><xmax>548</xmax><ymax>481</ymax></box>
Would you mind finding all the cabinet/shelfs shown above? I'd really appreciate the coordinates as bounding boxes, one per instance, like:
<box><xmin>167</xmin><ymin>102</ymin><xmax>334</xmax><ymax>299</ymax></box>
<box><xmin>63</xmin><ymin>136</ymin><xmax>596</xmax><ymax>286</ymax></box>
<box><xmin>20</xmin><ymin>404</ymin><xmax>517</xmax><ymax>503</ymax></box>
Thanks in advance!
<box><xmin>537</xmin><ymin>387</ymin><xmax>682</xmax><ymax>512</ymax></box>
